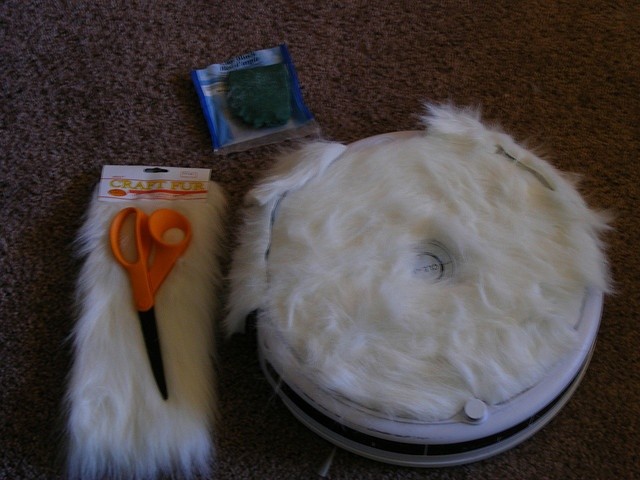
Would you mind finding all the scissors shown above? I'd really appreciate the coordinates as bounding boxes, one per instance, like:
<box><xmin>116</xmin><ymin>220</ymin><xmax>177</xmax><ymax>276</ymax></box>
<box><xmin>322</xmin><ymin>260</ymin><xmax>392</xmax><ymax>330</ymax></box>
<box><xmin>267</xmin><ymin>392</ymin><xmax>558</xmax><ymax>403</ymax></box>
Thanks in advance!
<box><xmin>110</xmin><ymin>209</ymin><xmax>193</xmax><ymax>401</ymax></box>
